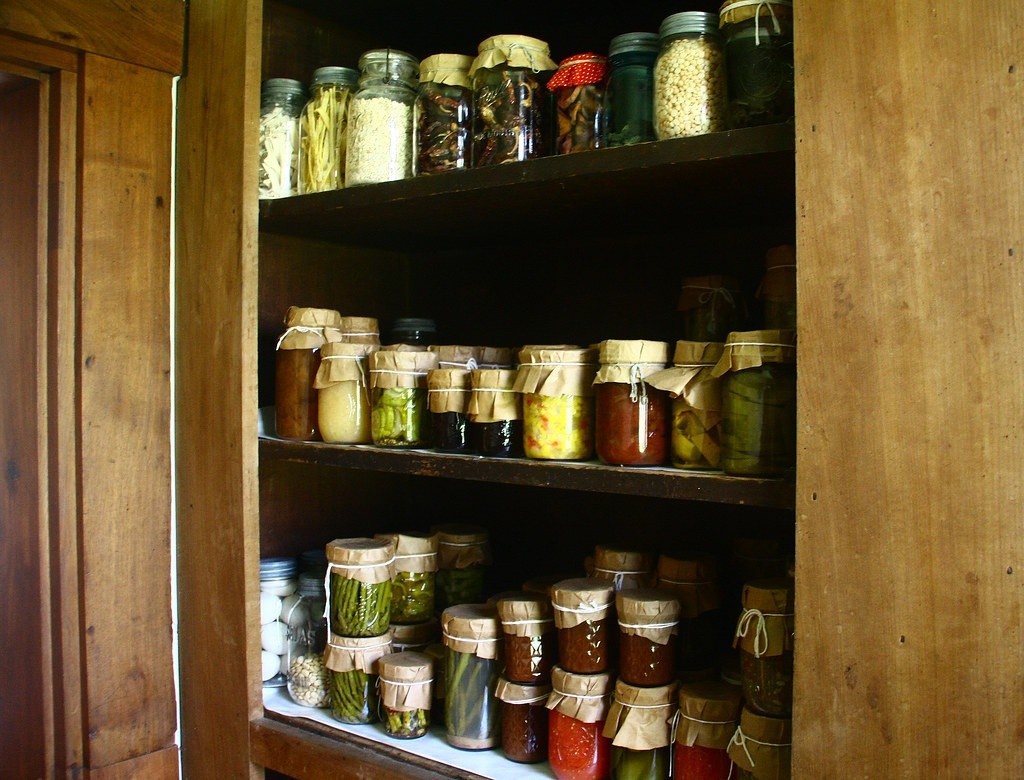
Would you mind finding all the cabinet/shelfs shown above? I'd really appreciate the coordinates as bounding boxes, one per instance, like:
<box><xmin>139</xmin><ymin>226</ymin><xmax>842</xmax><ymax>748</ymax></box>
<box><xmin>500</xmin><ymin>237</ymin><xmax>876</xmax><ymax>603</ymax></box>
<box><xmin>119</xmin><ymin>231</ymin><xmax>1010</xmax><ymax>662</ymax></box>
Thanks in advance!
<box><xmin>178</xmin><ymin>0</ymin><xmax>1023</xmax><ymax>780</ymax></box>
